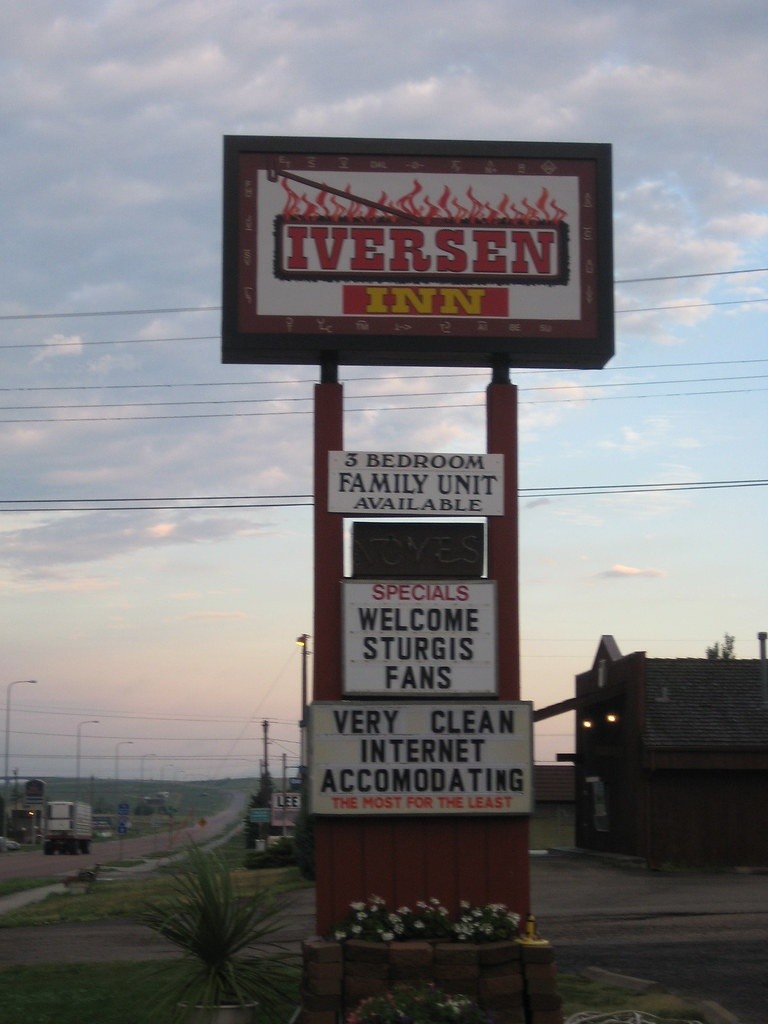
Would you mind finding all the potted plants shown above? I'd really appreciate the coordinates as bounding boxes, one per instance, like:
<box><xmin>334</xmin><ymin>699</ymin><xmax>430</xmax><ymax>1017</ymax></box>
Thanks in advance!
<box><xmin>130</xmin><ymin>833</ymin><xmax>297</xmax><ymax>1024</ymax></box>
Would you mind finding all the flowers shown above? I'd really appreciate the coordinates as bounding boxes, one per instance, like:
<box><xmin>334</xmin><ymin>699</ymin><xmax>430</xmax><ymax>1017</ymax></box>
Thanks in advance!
<box><xmin>345</xmin><ymin>983</ymin><xmax>478</xmax><ymax>1024</ymax></box>
<box><xmin>328</xmin><ymin>892</ymin><xmax>523</xmax><ymax>942</ymax></box>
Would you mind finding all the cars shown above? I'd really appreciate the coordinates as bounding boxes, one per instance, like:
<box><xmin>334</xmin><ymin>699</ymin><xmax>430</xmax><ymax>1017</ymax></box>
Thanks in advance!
<box><xmin>0</xmin><ymin>836</ymin><xmax>23</xmax><ymax>853</ymax></box>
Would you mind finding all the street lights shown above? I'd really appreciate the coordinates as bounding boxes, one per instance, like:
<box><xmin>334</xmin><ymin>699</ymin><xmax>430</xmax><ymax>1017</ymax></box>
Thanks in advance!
<box><xmin>115</xmin><ymin>741</ymin><xmax>134</xmax><ymax>806</ymax></box>
<box><xmin>138</xmin><ymin>753</ymin><xmax>157</xmax><ymax>801</ymax></box>
<box><xmin>74</xmin><ymin>720</ymin><xmax>100</xmax><ymax>801</ymax></box>
<box><xmin>295</xmin><ymin>633</ymin><xmax>314</xmax><ymax>710</ymax></box>
<box><xmin>4</xmin><ymin>679</ymin><xmax>38</xmax><ymax>792</ymax></box>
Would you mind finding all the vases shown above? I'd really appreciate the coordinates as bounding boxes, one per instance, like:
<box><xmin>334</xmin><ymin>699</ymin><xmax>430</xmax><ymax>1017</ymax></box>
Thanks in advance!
<box><xmin>300</xmin><ymin>935</ymin><xmax>562</xmax><ymax>1024</ymax></box>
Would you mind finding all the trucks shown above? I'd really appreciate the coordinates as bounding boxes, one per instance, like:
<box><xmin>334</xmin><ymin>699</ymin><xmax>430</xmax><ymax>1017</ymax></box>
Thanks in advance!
<box><xmin>39</xmin><ymin>800</ymin><xmax>100</xmax><ymax>855</ymax></box>
